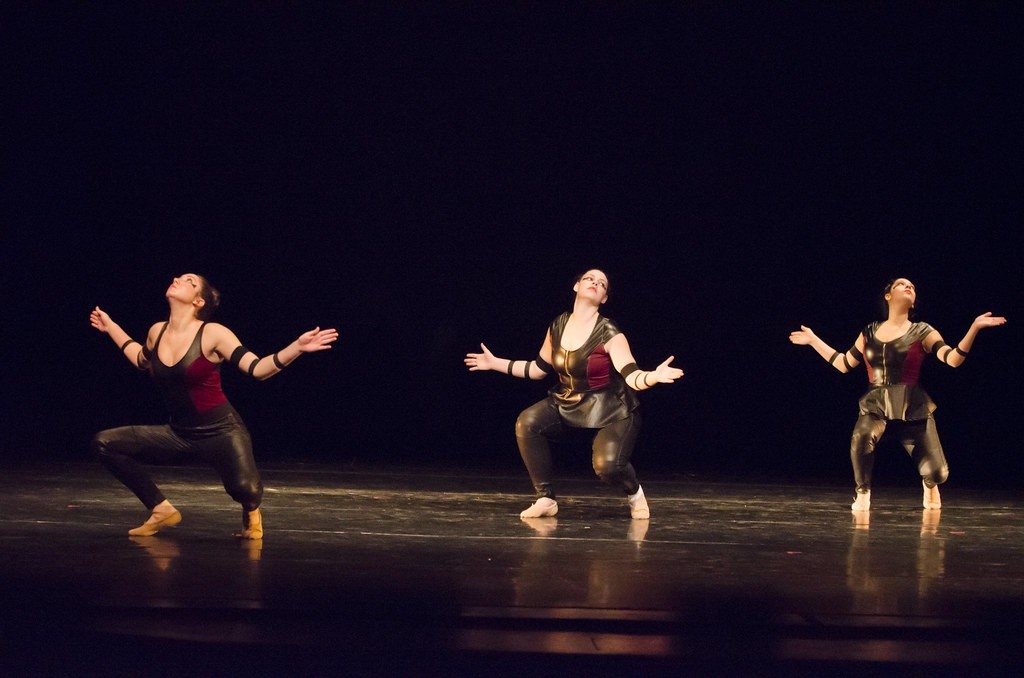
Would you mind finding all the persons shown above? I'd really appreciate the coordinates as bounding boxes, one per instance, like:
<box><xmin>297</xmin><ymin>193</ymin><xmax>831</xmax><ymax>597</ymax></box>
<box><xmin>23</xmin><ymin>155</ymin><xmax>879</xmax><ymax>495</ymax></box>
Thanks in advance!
<box><xmin>788</xmin><ymin>278</ymin><xmax>1007</xmax><ymax>510</ymax></box>
<box><xmin>88</xmin><ymin>272</ymin><xmax>339</xmax><ymax>539</ymax></box>
<box><xmin>464</xmin><ymin>268</ymin><xmax>683</xmax><ymax>519</ymax></box>
